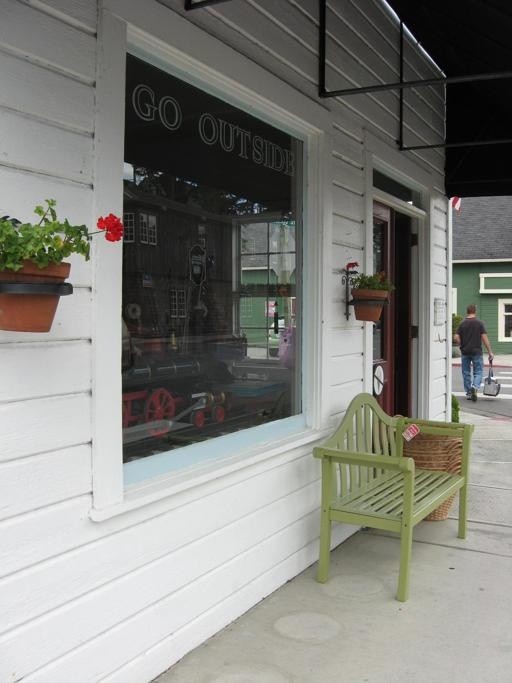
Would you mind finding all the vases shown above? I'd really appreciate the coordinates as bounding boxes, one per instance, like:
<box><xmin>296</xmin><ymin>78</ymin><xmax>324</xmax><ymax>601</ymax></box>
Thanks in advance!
<box><xmin>350</xmin><ymin>287</ymin><xmax>388</xmax><ymax>321</ymax></box>
<box><xmin>2</xmin><ymin>261</ymin><xmax>72</xmax><ymax>331</ymax></box>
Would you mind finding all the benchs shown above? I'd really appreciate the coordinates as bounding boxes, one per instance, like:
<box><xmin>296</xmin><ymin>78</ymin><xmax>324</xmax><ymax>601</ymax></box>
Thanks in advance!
<box><xmin>311</xmin><ymin>392</ymin><xmax>475</xmax><ymax>602</ymax></box>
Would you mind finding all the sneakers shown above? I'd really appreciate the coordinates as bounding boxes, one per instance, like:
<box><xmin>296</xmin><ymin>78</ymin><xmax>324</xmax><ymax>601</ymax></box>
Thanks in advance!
<box><xmin>466</xmin><ymin>385</ymin><xmax>477</xmax><ymax>401</ymax></box>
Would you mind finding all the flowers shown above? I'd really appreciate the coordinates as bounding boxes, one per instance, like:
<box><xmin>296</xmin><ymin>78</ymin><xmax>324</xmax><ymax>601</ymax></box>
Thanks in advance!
<box><xmin>345</xmin><ymin>261</ymin><xmax>396</xmax><ymax>291</ymax></box>
<box><xmin>1</xmin><ymin>197</ymin><xmax>123</xmax><ymax>273</ymax></box>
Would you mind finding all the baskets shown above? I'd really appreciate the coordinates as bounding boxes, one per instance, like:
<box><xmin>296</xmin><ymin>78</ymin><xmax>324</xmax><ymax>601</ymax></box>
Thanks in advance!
<box><xmin>404</xmin><ymin>433</ymin><xmax>462</xmax><ymax>521</ymax></box>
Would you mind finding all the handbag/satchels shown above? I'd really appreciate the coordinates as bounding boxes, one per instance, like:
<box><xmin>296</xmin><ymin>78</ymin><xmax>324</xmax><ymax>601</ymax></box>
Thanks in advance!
<box><xmin>484</xmin><ymin>377</ymin><xmax>500</xmax><ymax>396</ymax></box>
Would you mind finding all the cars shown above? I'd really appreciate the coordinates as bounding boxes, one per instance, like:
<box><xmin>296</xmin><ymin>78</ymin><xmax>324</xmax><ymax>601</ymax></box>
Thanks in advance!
<box><xmin>267</xmin><ymin>316</ymin><xmax>300</xmax><ymax>356</ymax></box>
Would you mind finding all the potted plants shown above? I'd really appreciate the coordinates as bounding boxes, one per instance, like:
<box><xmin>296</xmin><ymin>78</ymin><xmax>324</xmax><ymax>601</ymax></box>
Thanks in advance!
<box><xmin>452</xmin><ymin>312</ymin><xmax>465</xmax><ymax>357</ymax></box>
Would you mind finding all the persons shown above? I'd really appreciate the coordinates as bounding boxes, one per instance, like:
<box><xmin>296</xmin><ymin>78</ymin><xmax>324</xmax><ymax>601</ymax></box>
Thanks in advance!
<box><xmin>453</xmin><ymin>303</ymin><xmax>494</xmax><ymax>402</ymax></box>
